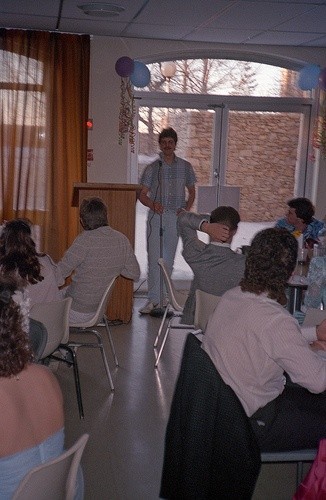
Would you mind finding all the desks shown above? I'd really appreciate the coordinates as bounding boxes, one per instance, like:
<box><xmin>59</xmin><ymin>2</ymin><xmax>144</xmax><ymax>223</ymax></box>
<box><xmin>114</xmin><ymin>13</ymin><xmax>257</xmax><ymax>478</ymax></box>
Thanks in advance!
<box><xmin>243</xmin><ymin>245</ymin><xmax>313</xmax><ymax>315</ymax></box>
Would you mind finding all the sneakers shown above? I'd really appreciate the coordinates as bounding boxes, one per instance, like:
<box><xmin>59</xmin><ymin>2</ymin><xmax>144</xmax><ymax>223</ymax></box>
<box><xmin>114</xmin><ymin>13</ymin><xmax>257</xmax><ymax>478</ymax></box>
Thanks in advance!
<box><xmin>139</xmin><ymin>303</ymin><xmax>161</xmax><ymax>314</ymax></box>
<box><xmin>163</xmin><ymin>303</ymin><xmax>175</xmax><ymax>311</ymax></box>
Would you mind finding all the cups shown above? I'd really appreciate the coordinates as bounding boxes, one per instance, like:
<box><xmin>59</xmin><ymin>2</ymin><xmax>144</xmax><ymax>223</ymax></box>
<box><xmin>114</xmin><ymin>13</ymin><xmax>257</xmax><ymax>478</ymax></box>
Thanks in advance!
<box><xmin>293</xmin><ymin>264</ymin><xmax>303</xmax><ymax>282</ymax></box>
<box><xmin>308</xmin><ymin>249</ymin><xmax>325</xmax><ymax>259</ymax></box>
<box><xmin>241</xmin><ymin>238</ymin><xmax>250</xmax><ymax>255</ymax></box>
<box><xmin>298</xmin><ymin>249</ymin><xmax>307</xmax><ymax>264</ymax></box>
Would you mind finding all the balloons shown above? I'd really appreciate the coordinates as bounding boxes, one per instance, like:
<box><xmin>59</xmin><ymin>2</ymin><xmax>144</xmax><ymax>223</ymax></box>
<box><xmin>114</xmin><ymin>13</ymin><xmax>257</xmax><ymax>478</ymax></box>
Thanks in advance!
<box><xmin>298</xmin><ymin>64</ymin><xmax>320</xmax><ymax>90</ymax></box>
<box><xmin>115</xmin><ymin>56</ymin><xmax>135</xmax><ymax>77</ymax></box>
<box><xmin>130</xmin><ymin>61</ymin><xmax>151</xmax><ymax>88</ymax></box>
<box><xmin>317</xmin><ymin>69</ymin><xmax>326</xmax><ymax>91</ymax></box>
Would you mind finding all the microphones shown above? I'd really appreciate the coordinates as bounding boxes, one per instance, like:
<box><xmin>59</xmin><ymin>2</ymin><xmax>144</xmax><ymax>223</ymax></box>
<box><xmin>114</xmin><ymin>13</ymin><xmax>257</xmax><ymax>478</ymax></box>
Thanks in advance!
<box><xmin>159</xmin><ymin>152</ymin><xmax>165</xmax><ymax>164</ymax></box>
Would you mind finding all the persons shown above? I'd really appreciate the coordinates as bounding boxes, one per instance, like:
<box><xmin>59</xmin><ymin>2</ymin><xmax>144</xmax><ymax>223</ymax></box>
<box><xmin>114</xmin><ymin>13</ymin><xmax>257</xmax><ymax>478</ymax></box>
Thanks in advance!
<box><xmin>0</xmin><ymin>218</ymin><xmax>65</xmax><ymax>319</ymax></box>
<box><xmin>56</xmin><ymin>196</ymin><xmax>141</xmax><ymax>326</ymax></box>
<box><xmin>200</xmin><ymin>226</ymin><xmax>325</xmax><ymax>439</ymax></box>
<box><xmin>0</xmin><ymin>273</ymin><xmax>64</xmax><ymax>500</ymax></box>
<box><xmin>138</xmin><ymin>126</ymin><xmax>196</xmax><ymax>314</ymax></box>
<box><xmin>276</xmin><ymin>196</ymin><xmax>326</xmax><ymax>247</ymax></box>
<box><xmin>177</xmin><ymin>206</ymin><xmax>248</xmax><ymax>324</ymax></box>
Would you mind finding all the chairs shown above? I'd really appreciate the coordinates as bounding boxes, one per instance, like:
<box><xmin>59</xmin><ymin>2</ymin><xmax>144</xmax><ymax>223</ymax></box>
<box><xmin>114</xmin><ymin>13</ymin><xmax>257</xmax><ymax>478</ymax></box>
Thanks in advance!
<box><xmin>11</xmin><ymin>433</ymin><xmax>90</xmax><ymax>500</ymax></box>
<box><xmin>153</xmin><ymin>258</ymin><xmax>190</xmax><ymax>368</ymax></box>
<box><xmin>193</xmin><ymin>288</ymin><xmax>223</xmax><ymax>334</ymax></box>
<box><xmin>68</xmin><ymin>275</ymin><xmax>118</xmax><ymax>391</ymax></box>
<box><xmin>29</xmin><ymin>297</ymin><xmax>84</xmax><ymax>421</ymax></box>
<box><xmin>158</xmin><ymin>332</ymin><xmax>319</xmax><ymax>499</ymax></box>
<box><xmin>26</xmin><ymin>317</ymin><xmax>47</xmax><ymax>364</ymax></box>
